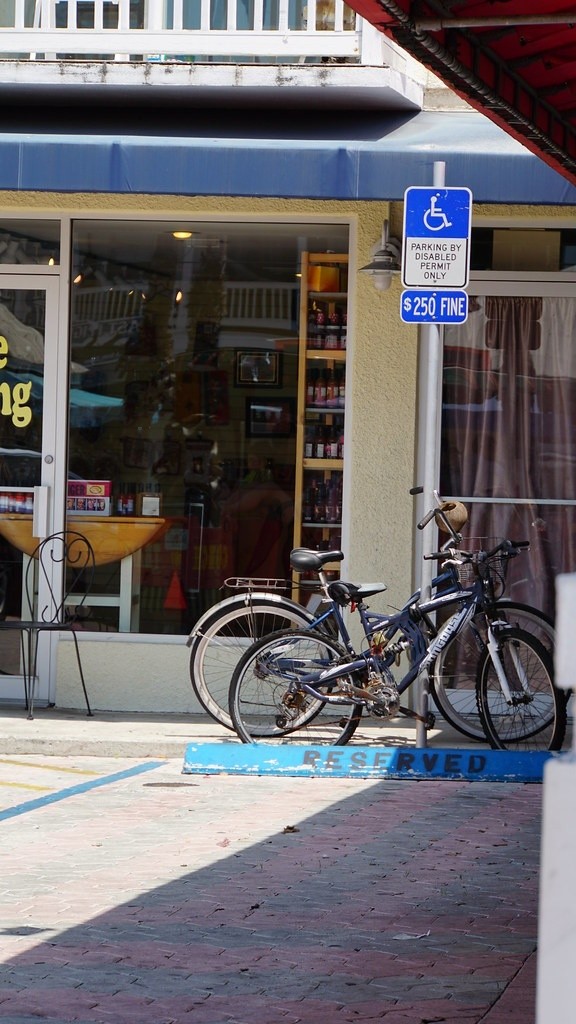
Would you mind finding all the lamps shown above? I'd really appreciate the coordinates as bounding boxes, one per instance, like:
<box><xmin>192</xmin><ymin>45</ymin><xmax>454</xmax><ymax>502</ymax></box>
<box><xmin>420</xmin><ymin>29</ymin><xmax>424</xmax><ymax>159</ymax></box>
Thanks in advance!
<box><xmin>37</xmin><ymin>255</ymin><xmax>54</xmax><ymax>266</ymax></box>
<box><xmin>72</xmin><ymin>266</ymin><xmax>165</xmax><ymax>300</ymax></box>
<box><xmin>357</xmin><ymin>219</ymin><xmax>401</xmax><ymax>291</ymax></box>
<box><xmin>0</xmin><ymin>233</ymin><xmax>36</xmax><ymax>264</ymax></box>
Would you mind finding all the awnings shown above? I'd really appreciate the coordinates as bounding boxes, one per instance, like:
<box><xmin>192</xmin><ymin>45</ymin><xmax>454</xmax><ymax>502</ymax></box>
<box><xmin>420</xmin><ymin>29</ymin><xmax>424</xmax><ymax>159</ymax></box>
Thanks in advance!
<box><xmin>0</xmin><ymin>371</ymin><xmax>125</xmax><ymax>428</ymax></box>
<box><xmin>344</xmin><ymin>0</ymin><xmax>576</xmax><ymax>186</ymax></box>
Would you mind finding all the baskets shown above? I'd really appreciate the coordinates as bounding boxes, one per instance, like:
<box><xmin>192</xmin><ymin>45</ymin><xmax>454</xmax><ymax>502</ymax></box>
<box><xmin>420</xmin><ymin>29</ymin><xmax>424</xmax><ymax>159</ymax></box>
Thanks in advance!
<box><xmin>458</xmin><ymin>536</ymin><xmax>510</xmax><ymax>583</ymax></box>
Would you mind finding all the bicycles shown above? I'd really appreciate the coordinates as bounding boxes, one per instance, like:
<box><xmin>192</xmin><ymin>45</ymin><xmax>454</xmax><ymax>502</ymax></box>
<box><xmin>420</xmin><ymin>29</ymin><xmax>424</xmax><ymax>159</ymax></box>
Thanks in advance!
<box><xmin>228</xmin><ymin>539</ymin><xmax>567</xmax><ymax>752</ymax></box>
<box><xmin>186</xmin><ymin>487</ymin><xmax>572</xmax><ymax>745</ymax></box>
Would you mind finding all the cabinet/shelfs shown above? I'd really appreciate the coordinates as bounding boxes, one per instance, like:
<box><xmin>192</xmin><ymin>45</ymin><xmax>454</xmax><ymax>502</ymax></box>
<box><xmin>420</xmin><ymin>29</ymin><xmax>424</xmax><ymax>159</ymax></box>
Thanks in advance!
<box><xmin>289</xmin><ymin>251</ymin><xmax>349</xmax><ymax>631</ymax></box>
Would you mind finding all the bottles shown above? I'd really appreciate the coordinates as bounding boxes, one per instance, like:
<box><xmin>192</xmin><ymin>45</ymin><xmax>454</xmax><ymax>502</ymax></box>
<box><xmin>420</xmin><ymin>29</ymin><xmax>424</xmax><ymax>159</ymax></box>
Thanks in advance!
<box><xmin>304</xmin><ymin>423</ymin><xmax>344</xmax><ymax>460</ymax></box>
<box><xmin>303</xmin><ymin>535</ymin><xmax>340</xmax><ymax>580</ymax></box>
<box><xmin>304</xmin><ymin>472</ymin><xmax>341</xmax><ymax>522</ymax></box>
<box><xmin>0</xmin><ymin>477</ymin><xmax>37</xmax><ymax>514</ymax></box>
<box><xmin>117</xmin><ymin>481</ymin><xmax>164</xmax><ymax>518</ymax></box>
<box><xmin>265</xmin><ymin>457</ymin><xmax>275</xmax><ymax>483</ymax></box>
<box><xmin>308</xmin><ymin>302</ymin><xmax>346</xmax><ymax>350</ymax></box>
<box><xmin>308</xmin><ymin>367</ymin><xmax>345</xmax><ymax>409</ymax></box>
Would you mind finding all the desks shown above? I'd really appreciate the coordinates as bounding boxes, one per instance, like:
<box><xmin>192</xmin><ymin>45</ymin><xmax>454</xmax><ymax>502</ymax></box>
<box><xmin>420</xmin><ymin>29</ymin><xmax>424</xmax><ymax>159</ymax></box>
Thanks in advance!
<box><xmin>0</xmin><ymin>513</ymin><xmax>171</xmax><ymax>633</ymax></box>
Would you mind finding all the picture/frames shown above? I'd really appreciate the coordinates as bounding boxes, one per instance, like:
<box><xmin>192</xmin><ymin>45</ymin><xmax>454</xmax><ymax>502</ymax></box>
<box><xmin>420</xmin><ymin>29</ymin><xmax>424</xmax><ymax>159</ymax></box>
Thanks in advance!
<box><xmin>233</xmin><ymin>348</ymin><xmax>283</xmax><ymax>390</ymax></box>
<box><xmin>246</xmin><ymin>396</ymin><xmax>296</xmax><ymax>438</ymax></box>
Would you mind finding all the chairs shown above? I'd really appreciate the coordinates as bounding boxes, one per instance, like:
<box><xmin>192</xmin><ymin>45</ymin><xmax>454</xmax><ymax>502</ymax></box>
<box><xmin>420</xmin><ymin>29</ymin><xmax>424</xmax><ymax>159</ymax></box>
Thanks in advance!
<box><xmin>0</xmin><ymin>530</ymin><xmax>96</xmax><ymax>721</ymax></box>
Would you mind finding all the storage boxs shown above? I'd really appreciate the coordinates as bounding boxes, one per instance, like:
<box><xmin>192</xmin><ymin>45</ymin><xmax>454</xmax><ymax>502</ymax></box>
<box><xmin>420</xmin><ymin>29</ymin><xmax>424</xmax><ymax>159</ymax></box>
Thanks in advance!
<box><xmin>134</xmin><ymin>491</ymin><xmax>163</xmax><ymax>517</ymax></box>
